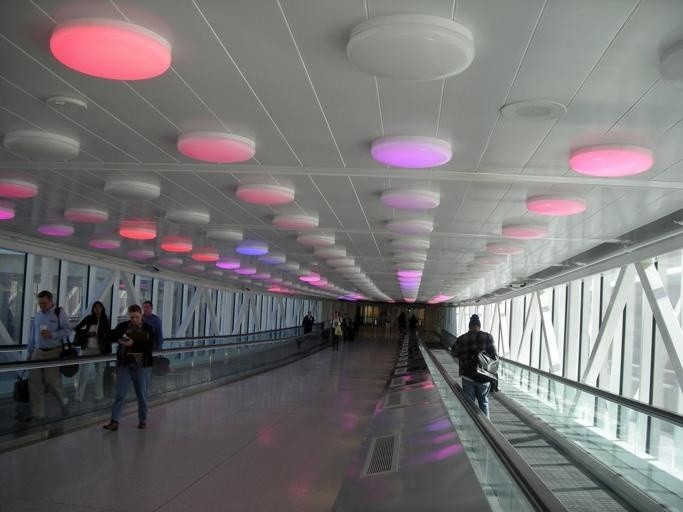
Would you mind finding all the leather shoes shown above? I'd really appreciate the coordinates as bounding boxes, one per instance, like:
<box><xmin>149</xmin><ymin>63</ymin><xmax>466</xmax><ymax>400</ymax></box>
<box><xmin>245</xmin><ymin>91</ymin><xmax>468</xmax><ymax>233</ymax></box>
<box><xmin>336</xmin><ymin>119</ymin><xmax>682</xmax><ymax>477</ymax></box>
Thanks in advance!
<box><xmin>138</xmin><ymin>421</ymin><xmax>146</xmax><ymax>428</ymax></box>
<box><xmin>102</xmin><ymin>422</ymin><xmax>119</xmax><ymax>430</ymax></box>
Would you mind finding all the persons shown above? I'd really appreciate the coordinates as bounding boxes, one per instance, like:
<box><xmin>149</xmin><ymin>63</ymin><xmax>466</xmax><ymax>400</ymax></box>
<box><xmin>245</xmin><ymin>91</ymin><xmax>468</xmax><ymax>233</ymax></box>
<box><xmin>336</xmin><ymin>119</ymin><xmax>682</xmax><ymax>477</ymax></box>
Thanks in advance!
<box><xmin>450</xmin><ymin>314</ymin><xmax>498</xmax><ymax>417</ymax></box>
<box><xmin>24</xmin><ymin>290</ymin><xmax>72</xmax><ymax>422</ymax></box>
<box><xmin>409</xmin><ymin>314</ymin><xmax>418</xmax><ymax>336</ymax></box>
<box><xmin>103</xmin><ymin>305</ymin><xmax>155</xmax><ymax>432</ymax></box>
<box><xmin>398</xmin><ymin>312</ymin><xmax>407</xmax><ymax>334</ymax></box>
<box><xmin>73</xmin><ymin>301</ymin><xmax>114</xmax><ymax>404</ymax></box>
<box><xmin>330</xmin><ymin>311</ymin><xmax>363</xmax><ymax>351</ymax></box>
<box><xmin>141</xmin><ymin>300</ymin><xmax>163</xmax><ymax>352</ymax></box>
<box><xmin>302</xmin><ymin>311</ymin><xmax>314</xmax><ymax>334</ymax></box>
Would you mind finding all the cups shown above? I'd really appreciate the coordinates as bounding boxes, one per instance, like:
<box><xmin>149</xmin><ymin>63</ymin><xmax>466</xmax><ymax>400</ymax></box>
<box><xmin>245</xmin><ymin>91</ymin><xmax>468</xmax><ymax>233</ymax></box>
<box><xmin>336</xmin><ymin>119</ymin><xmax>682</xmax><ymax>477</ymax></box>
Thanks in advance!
<box><xmin>40</xmin><ymin>324</ymin><xmax>47</xmax><ymax>331</ymax></box>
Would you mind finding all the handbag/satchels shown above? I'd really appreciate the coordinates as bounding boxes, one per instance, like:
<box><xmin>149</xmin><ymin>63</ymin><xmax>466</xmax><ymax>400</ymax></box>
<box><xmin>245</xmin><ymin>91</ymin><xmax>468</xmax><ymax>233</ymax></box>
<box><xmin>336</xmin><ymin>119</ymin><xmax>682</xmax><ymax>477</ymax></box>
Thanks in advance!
<box><xmin>59</xmin><ymin>349</ymin><xmax>79</xmax><ymax>376</ymax></box>
<box><xmin>335</xmin><ymin>326</ymin><xmax>343</xmax><ymax>336</ymax></box>
<box><xmin>476</xmin><ymin>351</ymin><xmax>498</xmax><ymax>381</ymax></box>
<box><xmin>14</xmin><ymin>380</ymin><xmax>29</xmax><ymax>402</ymax></box>
